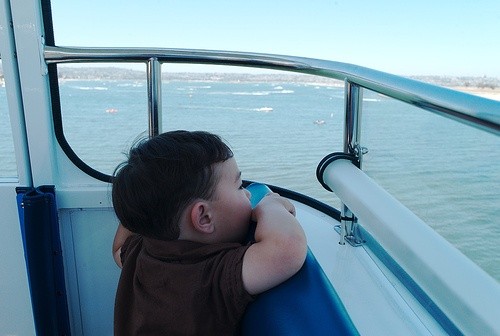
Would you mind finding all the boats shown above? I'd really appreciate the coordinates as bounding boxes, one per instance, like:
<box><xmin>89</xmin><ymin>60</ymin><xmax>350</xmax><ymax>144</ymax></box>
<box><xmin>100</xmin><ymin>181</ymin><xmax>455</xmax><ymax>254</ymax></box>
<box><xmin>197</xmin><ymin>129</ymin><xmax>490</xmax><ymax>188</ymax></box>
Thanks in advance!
<box><xmin>106</xmin><ymin>108</ymin><xmax>118</xmax><ymax>113</ymax></box>
<box><xmin>256</xmin><ymin>106</ymin><xmax>274</xmax><ymax>112</ymax></box>
<box><xmin>246</xmin><ymin>80</ymin><xmax>283</xmax><ymax>91</ymax></box>
<box><xmin>312</xmin><ymin>118</ymin><xmax>325</xmax><ymax>125</ymax></box>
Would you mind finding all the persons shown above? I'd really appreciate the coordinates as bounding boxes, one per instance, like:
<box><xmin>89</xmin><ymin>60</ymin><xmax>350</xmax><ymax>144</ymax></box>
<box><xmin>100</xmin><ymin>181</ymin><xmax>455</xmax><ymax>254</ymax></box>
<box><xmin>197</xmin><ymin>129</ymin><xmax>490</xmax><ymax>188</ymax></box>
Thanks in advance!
<box><xmin>110</xmin><ymin>130</ymin><xmax>308</xmax><ymax>336</ymax></box>
